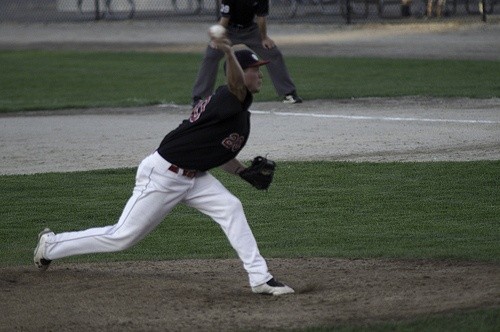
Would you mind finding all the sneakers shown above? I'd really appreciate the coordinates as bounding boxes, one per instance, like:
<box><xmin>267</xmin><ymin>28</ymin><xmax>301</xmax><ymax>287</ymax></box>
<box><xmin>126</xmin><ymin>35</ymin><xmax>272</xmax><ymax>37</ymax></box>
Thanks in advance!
<box><xmin>192</xmin><ymin>96</ymin><xmax>204</xmax><ymax>112</ymax></box>
<box><xmin>33</xmin><ymin>228</ymin><xmax>55</xmax><ymax>274</ymax></box>
<box><xmin>282</xmin><ymin>90</ymin><xmax>303</xmax><ymax>103</ymax></box>
<box><xmin>251</xmin><ymin>276</ymin><xmax>295</xmax><ymax>295</ymax></box>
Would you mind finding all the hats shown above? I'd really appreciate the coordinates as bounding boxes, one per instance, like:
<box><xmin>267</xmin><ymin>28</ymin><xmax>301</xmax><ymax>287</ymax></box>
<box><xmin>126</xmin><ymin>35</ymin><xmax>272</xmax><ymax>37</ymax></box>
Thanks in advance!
<box><xmin>224</xmin><ymin>50</ymin><xmax>270</xmax><ymax>71</ymax></box>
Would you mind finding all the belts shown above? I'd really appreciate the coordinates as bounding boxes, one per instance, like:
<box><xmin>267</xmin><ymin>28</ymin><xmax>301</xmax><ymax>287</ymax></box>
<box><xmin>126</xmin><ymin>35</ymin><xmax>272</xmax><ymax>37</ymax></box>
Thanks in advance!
<box><xmin>167</xmin><ymin>164</ymin><xmax>204</xmax><ymax>179</ymax></box>
<box><xmin>229</xmin><ymin>22</ymin><xmax>253</xmax><ymax>29</ymax></box>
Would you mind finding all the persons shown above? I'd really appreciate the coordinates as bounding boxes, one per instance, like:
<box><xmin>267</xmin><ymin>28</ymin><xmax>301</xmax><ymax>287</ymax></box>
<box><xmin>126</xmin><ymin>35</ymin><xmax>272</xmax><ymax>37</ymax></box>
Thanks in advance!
<box><xmin>33</xmin><ymin>38</ymin><xmax>296</xmax><ymax>296</ymax></box>
<box><xmin>193</xmin><ymin>0</ymin><xmax>302</xmax><ymax>104</ymax></box>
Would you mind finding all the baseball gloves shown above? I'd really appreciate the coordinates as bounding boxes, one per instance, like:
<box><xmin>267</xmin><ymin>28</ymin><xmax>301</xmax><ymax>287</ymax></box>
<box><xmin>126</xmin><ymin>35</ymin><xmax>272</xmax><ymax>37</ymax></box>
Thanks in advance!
<box><xmin>239</xmin><ymin>155</ymin><xmax>275</xmax><ymax>191</ymax></box>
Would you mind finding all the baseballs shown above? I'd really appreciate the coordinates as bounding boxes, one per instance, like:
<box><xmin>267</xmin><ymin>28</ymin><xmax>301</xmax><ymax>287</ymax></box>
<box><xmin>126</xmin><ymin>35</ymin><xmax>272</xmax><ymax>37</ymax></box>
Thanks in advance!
<box><xmin>209</xmin><ymin>25</ymin><xmax>226</xmax><ymax>39</ymax></box>
